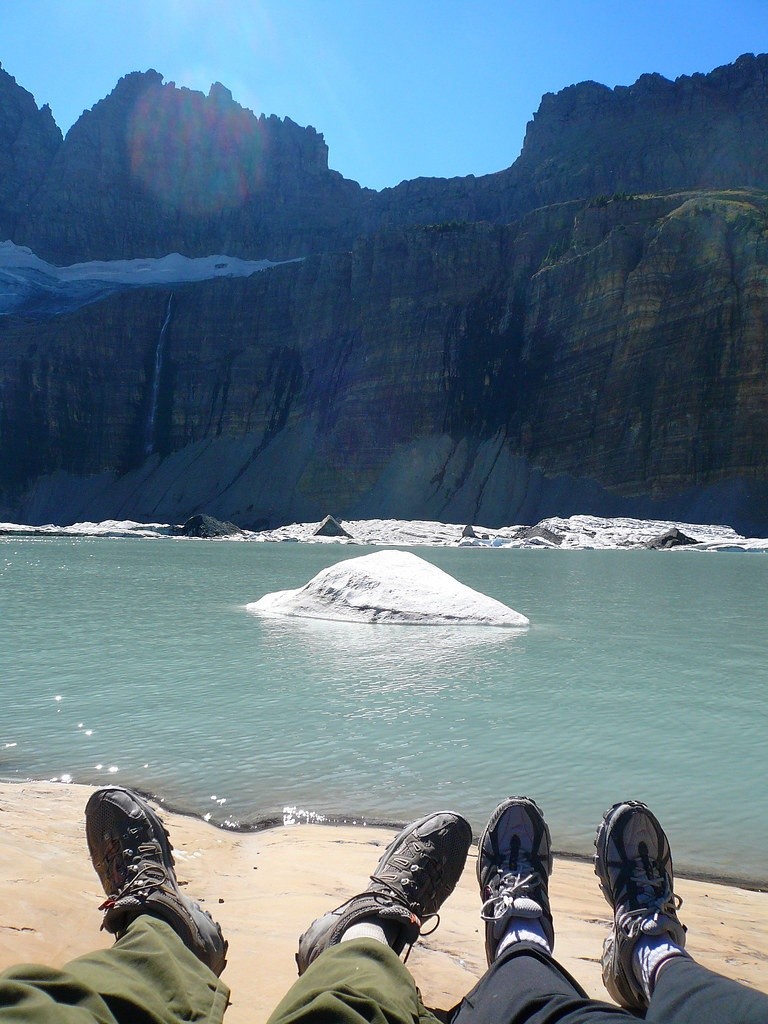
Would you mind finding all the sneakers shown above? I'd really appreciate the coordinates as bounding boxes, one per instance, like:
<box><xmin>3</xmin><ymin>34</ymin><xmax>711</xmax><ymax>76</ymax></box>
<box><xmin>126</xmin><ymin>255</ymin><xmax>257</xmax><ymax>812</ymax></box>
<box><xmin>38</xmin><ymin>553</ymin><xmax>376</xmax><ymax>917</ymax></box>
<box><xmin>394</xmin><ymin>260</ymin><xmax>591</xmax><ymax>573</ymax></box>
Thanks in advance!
<box><xmin>476</xmin><ymin>796</ymin><xmax>556</xmax><ymax>967</ymax></box>
<box><xmin>296</xmin><ymin>811</ymin><xmax>473</xmax><ymax>986</ymax></box>
<box><xmin>85</xmin><ymin>788</ymin><xmax>229</xmax><ymax>981</ymax></box>
<box><xmin>594</xmin><ymin>801</ymin><xmax>687</xmax><ymax>1012</ymax></box>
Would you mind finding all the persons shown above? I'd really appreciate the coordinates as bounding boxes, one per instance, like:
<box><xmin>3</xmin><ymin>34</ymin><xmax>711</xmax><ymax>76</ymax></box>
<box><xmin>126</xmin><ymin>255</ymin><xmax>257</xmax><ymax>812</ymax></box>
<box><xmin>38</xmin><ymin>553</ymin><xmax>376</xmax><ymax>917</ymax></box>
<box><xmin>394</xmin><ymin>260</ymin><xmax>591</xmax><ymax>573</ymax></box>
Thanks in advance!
<box><xmin>447</xmin><ymin>795</ymin><xmax>768</xmax><ymax>1024</ymax></box>
<box><xmin>0</xmin><ymin>784</ymin><xmax>473</xmax><ymax>1024</ymax></box>
